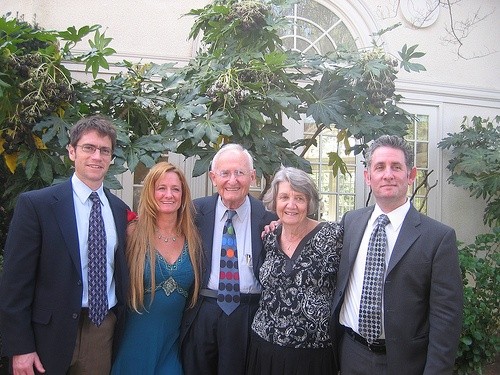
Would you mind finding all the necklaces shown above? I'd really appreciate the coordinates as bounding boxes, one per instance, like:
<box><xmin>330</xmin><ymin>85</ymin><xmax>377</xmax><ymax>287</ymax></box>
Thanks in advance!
<box><xmin>283</xmin><ymin>231</ymin><xmax>304</xmax><ymax>250</ymax></box>
<box><xmin>154</xmin><ymin>229</ymin><xmax>180</xmax><ymax>242</ymax></box>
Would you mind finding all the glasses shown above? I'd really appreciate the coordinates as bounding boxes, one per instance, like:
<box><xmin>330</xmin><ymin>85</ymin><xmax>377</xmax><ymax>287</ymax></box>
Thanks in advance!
<box><xmin>74</xmin><ymin>144</ymin><xmax>114</xmax><ymax>156</ymax></box>
<box><xmin>214</xmin><ymin>170</ymin><xmax>253</xmax><ymax>178</ymax></box>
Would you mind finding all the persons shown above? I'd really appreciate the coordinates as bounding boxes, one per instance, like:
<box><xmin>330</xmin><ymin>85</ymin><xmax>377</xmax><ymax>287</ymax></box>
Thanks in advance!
<box><xmin>245</xmin><ymin>167</ymin><xmax>345</xmax><ymax>375</ymax></box>
<box><xmin>189</xmin><ymin>143</ymin><xmax>280</xmax><ymax>375</ymax></box>
<box><xmin>111</xmin><ymin>162</ymin><xmax>204</xmax><ymax>375</ymax></box>
<box><xmin>260</xmin><ymin>135</ymin><xmax>464</xmax><ymax>375</ymax></box>
<box><xmin>0</xmin><ymin>117</ymin><xmax>136</xmax><ymax>375</ymax></box>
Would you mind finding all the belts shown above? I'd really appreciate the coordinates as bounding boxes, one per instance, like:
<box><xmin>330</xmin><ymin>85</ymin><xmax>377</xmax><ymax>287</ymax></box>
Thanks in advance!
<box><xmin>200</xmin><ymin>288</ymin><xmax>259</xmax><ymax>303</ymax></box>
<box><xmin>344</xmin><ymin>326</ymin><xmax>387</xmax><ymax>354</ymax></box>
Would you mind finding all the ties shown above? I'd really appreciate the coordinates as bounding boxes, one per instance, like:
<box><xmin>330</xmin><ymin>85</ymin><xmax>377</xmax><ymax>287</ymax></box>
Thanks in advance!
<box><xmin>217</xmin><ymin>210</ymin><xmax>241</xmax><ymax>316</ymax></box>
<box><xmin>88</xmin><ymin>192</ymin><xmax>108</xmax><ymax>329</ymax></box>
<box><xmin>359</xmin><ymin>214</ymin><xmax>390</xmax><ymax>346</ymax></box>
